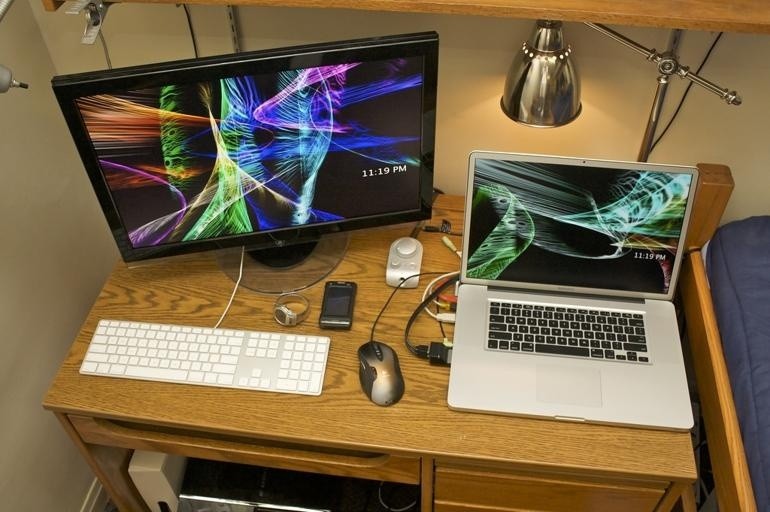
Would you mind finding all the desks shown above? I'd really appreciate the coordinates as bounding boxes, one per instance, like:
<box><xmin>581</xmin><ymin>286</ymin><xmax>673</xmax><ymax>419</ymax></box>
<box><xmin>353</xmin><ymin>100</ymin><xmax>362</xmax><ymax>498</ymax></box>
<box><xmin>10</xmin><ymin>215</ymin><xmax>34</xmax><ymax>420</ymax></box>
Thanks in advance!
<box><xmin>39</xmin><ymin>191</ymin><xmax>699</xmax><ymax>510</ymax></box>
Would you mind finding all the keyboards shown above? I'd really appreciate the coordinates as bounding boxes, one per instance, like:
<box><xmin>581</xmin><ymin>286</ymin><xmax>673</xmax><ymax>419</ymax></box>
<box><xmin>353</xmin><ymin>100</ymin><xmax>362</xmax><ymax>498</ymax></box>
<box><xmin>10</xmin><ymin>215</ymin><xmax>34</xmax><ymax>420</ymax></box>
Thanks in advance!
<box><xmin>78</xmin><ymin>319</ymin><xmax>331</xmax><ymax>397</ymax></box>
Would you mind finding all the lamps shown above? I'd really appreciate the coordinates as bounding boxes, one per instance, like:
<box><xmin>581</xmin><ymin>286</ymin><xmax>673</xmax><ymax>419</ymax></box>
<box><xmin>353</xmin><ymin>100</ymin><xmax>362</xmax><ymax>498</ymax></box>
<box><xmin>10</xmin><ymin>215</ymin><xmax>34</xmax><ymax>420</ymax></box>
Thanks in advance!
<box><xmin>498</xmin><ymin>19</ymin><xmax>744</xmax><ymax>167</ymax></box>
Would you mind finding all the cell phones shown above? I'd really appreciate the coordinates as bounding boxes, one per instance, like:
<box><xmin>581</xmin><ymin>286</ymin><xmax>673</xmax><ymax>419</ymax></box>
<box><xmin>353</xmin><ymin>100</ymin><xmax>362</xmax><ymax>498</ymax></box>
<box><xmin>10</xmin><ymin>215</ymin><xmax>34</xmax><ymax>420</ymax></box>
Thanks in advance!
<box><xmin>319</xmin><ymin>281</ymin><xmax>357</xmax><ymax>329</ymax></box>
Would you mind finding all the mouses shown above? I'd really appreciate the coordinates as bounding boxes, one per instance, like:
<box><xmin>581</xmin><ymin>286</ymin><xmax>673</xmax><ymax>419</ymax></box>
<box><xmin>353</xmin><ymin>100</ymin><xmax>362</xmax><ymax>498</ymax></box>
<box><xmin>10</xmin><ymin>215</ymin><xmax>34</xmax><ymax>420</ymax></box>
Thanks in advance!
<box><xmin>357</xmin><ymin>341</ymin><xmax>405</xmax><ymax>407</ymax></box>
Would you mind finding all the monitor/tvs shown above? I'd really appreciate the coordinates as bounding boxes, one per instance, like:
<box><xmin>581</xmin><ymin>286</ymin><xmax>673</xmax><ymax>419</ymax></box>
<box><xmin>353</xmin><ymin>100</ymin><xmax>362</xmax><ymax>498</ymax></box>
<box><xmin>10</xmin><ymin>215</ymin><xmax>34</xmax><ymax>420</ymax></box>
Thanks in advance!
<box><xmin>51</xmin><ymin>30</ymin><xmax>440</xmax><ymax>295</ymax></box>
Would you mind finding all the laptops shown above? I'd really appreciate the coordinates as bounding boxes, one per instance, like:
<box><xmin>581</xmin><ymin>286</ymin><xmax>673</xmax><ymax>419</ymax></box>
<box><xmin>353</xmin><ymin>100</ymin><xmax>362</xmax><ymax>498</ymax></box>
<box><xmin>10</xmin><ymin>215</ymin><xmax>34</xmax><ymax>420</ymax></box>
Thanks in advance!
<box><xmin>447</xmin><ymin>151</ymin><xmax>701</xmax><ymax>434</ymax></box>
